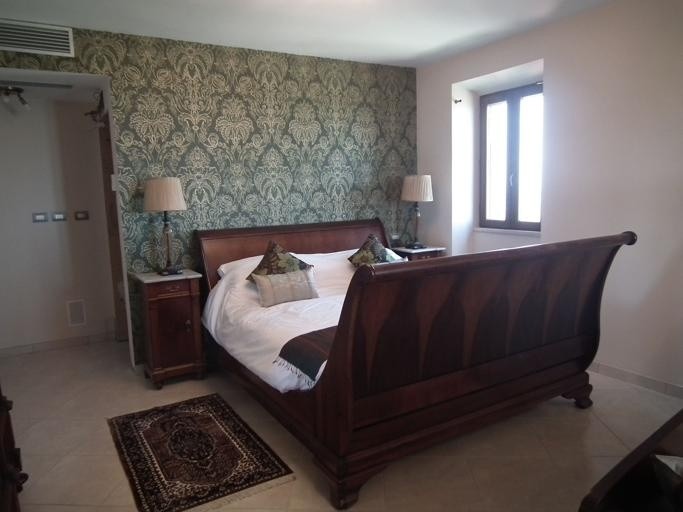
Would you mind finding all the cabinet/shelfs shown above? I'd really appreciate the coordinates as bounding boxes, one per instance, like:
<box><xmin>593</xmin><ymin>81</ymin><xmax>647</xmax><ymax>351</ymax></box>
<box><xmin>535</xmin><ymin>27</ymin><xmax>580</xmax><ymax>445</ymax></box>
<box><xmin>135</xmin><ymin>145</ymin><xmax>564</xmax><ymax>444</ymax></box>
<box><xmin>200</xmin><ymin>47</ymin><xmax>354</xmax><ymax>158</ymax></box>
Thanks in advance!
<box><xmin>1</xmin><ymin>395</ymin><xmax>30</xmax><ymax>510</ymax></box>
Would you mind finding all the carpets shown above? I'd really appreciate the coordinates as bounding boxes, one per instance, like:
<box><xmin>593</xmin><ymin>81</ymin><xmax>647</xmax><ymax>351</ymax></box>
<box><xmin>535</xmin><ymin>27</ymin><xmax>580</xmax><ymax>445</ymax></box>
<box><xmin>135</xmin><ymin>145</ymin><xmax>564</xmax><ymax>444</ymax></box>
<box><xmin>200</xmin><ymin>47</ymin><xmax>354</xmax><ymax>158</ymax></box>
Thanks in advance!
<box><xmin>106</xmin><ymin>390</ymin><xmax>300</xmax><ymax>512</ymax></box>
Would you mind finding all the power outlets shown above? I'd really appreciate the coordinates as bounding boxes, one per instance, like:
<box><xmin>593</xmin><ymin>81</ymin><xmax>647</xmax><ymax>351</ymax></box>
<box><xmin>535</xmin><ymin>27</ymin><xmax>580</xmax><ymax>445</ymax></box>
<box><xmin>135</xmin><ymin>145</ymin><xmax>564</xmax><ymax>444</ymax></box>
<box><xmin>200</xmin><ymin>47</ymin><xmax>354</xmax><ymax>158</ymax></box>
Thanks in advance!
<box><xmin>392</xmin><ymin>234</ymin><xmax>399</xmax><ymax>241</ymax></box>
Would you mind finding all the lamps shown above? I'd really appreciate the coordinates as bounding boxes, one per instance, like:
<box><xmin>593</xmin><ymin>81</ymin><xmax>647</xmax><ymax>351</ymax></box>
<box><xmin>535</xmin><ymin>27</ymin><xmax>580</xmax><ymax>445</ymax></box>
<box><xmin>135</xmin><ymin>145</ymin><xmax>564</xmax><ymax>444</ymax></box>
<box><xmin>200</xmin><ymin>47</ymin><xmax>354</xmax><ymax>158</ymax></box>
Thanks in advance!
<box><xmin>142</xmin><ymin>177</ymin><xmax>187</xmax><ymax>275</ymax></box>
<box><xmin>400</xmin><ymin>174</ymin><xmax>434</xmax><ymax>249</ymax></box>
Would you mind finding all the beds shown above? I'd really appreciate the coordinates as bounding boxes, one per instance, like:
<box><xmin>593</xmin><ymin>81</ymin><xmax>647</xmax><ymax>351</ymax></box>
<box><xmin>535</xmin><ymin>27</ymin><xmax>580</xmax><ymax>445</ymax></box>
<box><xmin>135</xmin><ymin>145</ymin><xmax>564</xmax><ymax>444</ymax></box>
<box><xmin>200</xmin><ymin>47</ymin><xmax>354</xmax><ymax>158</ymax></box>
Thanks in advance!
<box><xmin>193</xmin><ymin>215</ymin><xmax>640</xmax><ymax>505</ymax></box>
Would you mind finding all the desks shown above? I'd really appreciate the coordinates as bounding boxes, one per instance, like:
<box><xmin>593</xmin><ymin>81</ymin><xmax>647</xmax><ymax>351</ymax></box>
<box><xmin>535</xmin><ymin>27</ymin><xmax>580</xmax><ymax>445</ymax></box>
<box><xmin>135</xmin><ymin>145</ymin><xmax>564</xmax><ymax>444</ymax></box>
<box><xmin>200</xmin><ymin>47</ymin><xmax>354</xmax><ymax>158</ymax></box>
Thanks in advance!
<box><xmin>577</xmin><ymin>405</ymin><xmax>683</xmax><ymax>510</ymax></box>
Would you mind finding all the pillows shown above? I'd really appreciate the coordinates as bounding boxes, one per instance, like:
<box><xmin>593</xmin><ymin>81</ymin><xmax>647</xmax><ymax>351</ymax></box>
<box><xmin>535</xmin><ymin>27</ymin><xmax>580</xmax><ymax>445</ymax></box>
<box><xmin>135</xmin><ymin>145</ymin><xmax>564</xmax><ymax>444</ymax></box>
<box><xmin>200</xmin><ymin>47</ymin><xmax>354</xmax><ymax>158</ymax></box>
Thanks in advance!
<box><xmin>345</xmin><ymin>233</ymin><xmax>398</xmax><ymax>267</ymax></box>
<box><xmin>245</xmin><ymin>238</ymin><xmax>315</xmax><ymax>280</ymax></box>
<box><xmin>251</xmin><ymin>266</ymin><xmax>320</xmax><ymax>307</ymax></box>
<box><xmin>215</xmin><ymin>250</ymin><xmax>302</xmax><ymax>278</ymax></box>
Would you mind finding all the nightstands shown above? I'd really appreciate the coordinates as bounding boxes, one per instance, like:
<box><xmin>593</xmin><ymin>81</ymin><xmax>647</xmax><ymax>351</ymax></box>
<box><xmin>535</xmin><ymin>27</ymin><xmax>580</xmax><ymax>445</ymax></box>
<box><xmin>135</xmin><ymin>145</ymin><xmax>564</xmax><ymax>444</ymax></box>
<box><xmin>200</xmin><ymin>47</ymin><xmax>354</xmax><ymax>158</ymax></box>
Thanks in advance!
<box><xmin>134</xmin><ymin>267</ymin><xmax>205</xmax><ymax>389</ymax></box>
<box><xmin>392</xmin><ymin>245</ymin><xmax>446</xmax><ymax>261</ymax></box>
